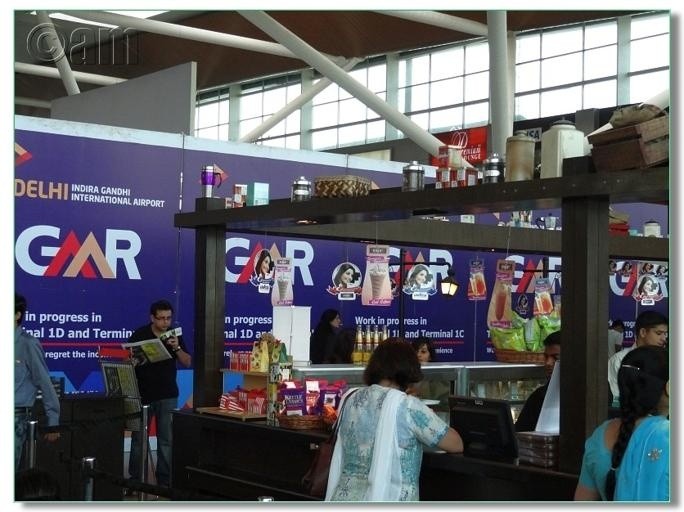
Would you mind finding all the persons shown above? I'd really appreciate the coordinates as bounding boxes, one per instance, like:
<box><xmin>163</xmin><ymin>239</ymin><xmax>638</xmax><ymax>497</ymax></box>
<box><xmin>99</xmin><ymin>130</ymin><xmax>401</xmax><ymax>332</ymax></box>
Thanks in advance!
<box><xmin>310</xmin><ymin>309</ymin><xmax>341</xmax><ymax>363</ymax></box>
<box><xmin>637</xmin><ymin>277</ymin><xmax>663</xmax><ymax>301</ymax></box>
<box><xmin>606</xmin><ymin>311</ymin><xmax>669</xmax><ymax>408</ymax></box>
<box><xmin>255</xmin><ymin>251</ymin><xmax>272</xmax><ymax>278</ymax></box>
<box><xmin>608</xmin><ymin>318</ymin><xmax>626</xmax><ymax>361</ymax></box>
<box><xmin>333</xmin><ymin>264</ymin><xmax>356</xmax><ymax>289</ymax></box>
<box><xmin>408</xmin><ymin>266</ymin><xmax>430</xmax><ymax>289</ymax></box>
<box><xmin>642</xmin><ymin>263</ymin><xmax>654</xmax><ymax>274</ymax></box>
<box><xmin>519</xmin><ymin>295</ymin><xmax>528</xmax><ymax>309</ymax></box>
<box><xmin>322</xmin><ymin>338</ymin><xmax>465</xmax><ymax>501</ymax></box>
<box><xmin>126</xmin><ymin>299</ymin><xmax>193</xmax><ymax>500</ymax></box>
<box><xmin>513</xmin><ymin>331</ymin><xmax>562</xmax><ymax>432</ymax></box>
<box><xmin>618</xmin><ymin>262</ymin><xmax>631</xmax><ymax>273</ymax></box>
<box><xmin>411</xmin><ymin>335</ymin><xmax>439</xmax><ymax>363</ymax></box>
<box><xmin>608</xmin><ymin>261</ymin><xmax>617</xmax><ymax>272</ymax></box>
<box><xmin>656</xmin><ymin>264</ymin><xmax>668</xmax><ymax>277</ymax></box>
<box><xmin>14</xmin><ymin>292</ymin><xmax>62</xmax><ymax>473</ymax></box>
<box><xmin>321</xmin><ymin>329</ymin><xmax>356</xmax><ymax>365</ymax></box>
<box><xmin>574</xmin><ymin>345</ymin><xmax>669</xmax><ymax>501</ymax></box>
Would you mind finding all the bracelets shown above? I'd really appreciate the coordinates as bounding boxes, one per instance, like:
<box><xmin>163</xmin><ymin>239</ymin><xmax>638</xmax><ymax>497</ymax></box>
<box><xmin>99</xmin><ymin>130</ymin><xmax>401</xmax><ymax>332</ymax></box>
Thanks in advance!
<box><xmin>171</xmin><ymin>346</ymin><xmax>181</xmax><ymax>353</ymax></box>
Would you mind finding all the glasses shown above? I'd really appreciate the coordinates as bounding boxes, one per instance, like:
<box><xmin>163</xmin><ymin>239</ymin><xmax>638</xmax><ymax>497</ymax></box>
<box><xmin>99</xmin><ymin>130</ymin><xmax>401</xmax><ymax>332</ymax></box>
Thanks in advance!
<box><xmin>154</xmin><ymin>316</ymin><xmax>171</xmax><ymax>322</ymax></box>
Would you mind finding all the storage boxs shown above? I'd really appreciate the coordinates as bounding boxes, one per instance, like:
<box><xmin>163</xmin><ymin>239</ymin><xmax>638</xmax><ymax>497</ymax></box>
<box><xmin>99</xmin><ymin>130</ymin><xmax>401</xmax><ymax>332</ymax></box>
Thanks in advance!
<box><xmin>586</xmin><ymin>110</ymin><xmax>669</xmax><ymax>172</ymax></box>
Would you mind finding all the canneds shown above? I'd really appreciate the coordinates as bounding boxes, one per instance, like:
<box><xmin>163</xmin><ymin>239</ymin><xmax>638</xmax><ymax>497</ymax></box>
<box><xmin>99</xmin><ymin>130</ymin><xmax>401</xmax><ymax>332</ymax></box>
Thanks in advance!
<box><xmin>434</xmin><ymin>145</ymin><xmax>478</xmax><ymax>190</ymax></box>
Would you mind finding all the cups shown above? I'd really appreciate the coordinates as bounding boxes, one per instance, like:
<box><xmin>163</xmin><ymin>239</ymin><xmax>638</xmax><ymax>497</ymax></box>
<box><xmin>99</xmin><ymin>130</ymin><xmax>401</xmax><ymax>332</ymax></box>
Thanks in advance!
<box><xmin>469</xmin><ymin>273</ymin><xmax>484</xmax><ymax>297</ymax></box>
<box><xmin>369</xmin><ymin>273</ymin><xmax>385</xmax><ymax>301</ymax></box>
<box><xmin>534</xmin><ymin>292</ymin><xmax>552</xmax><ymax>313</ymax></box>
<box><xmin>200</xmin><ymin>165</ymin><xmax>221</xmax><ymax>198</ymax></box>
<box><xmin>494</xmin><ymin>292</ymin><xmax>506</xmax><ymax>319</ymax></box>
<box><xmin>277</xmin><ymin>280</ymin><xmax>288</xmax><ymax>301</ymax></box>
<box><xmin>535</xmin><ymin>213</ymin><xmax>556</xmax><ymax>230</ymax></box>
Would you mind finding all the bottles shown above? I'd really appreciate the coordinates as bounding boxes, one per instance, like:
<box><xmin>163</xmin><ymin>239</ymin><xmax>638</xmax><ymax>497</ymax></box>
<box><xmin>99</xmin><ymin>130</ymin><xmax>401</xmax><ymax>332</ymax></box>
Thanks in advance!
<box><xmin>505</xmin><ymin>130</ymin><xmax>534</xmax><ymax>182</ymax></box>
<box><xmin>643</xmin><ymin>221</ymin><xmax>661</xmax><ymax>238</ymax></box>
<box><xmin>289</xmin><ymin>177</ymin><xmax>311</xmax><ymax>203</ymax></box>
<box><xmin>352</xmin><ymin>323</ymin><xmax>388</xmax><ymax>367</ymax></box>
<box><xmin>402</xmin><ymin>161</ymin><xmax>424</xmax><ymax>193</ymax></box>
<box><xmin>538</xmin><ymin>119</ymin><xmax>584</xmax><ymax>180</ymax></box>
<box><xmin>482</xmin><ymin>152</ymin><xmax>506</xmax><ymax>184</ymax></box>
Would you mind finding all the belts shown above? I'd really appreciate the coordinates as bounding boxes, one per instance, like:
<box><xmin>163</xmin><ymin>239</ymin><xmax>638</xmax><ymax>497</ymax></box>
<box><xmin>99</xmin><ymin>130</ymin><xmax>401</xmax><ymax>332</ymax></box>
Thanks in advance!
<box><xmin>16</xmin><ymin>407</ymin><xmax>33</xmax><ymax>414</ymax></box>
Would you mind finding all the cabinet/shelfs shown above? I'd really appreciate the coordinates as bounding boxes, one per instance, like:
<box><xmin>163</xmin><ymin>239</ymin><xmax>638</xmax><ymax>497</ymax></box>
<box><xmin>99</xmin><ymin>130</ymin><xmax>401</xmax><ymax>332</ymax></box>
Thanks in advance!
<box><xmin>32</xmin><ymin>399</ymin><xmax>126</xmax><ymax>501</ymax></box>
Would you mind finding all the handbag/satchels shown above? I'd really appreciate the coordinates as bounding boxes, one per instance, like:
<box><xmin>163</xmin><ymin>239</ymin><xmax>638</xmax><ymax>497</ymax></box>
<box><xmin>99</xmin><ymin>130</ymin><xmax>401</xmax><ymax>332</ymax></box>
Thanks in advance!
<box><xmin>300</xmin><ymin>389</ymin><xmax>358</xmax><ymax>498</ymax></box>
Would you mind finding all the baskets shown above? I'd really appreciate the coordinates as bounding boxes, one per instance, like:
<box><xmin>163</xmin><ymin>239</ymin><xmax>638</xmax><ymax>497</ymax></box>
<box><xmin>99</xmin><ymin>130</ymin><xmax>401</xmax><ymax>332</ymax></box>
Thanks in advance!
<box><xmin>275</xmin><ymin>413</ymin><xmax>324</xmax><ymax>429</ymax></box>
<box><xmin>315</xmin><ymin>176</ymin><xmax>370</xmax><ymax>198</ymax></box>
<box><xmin>494</xmin><ymin>348</ymin><xmax>545</xmax><ymax>365</ymax></box>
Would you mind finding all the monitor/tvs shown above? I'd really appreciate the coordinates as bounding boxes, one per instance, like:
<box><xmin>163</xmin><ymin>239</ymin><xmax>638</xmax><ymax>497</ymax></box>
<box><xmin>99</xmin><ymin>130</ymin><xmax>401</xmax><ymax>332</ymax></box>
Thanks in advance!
<box><xmin>448</xmin><ymin>395</ymin><xmax>518</xmax><ymax>464</ymax></box>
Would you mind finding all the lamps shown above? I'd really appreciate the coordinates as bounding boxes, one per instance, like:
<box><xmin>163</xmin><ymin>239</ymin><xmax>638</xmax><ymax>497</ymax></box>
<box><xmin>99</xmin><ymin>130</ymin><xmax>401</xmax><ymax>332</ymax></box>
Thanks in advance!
<box><xmin>404</xmin><ymin>261</ymin><xmax>459</xmax><ymax>298</ymax></box>
